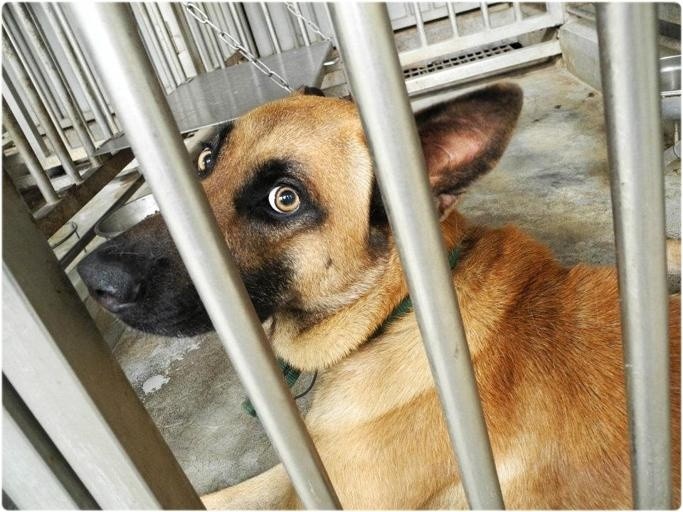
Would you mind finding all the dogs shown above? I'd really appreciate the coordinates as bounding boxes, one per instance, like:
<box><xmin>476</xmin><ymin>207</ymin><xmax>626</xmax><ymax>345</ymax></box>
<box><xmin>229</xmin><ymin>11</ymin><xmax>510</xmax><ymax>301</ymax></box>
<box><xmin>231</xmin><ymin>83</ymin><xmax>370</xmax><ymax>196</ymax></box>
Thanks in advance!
<box><xmin>75</xmin><ymin>82</ymin><xmax>681</xmax><ymax>509</ymax></box>
